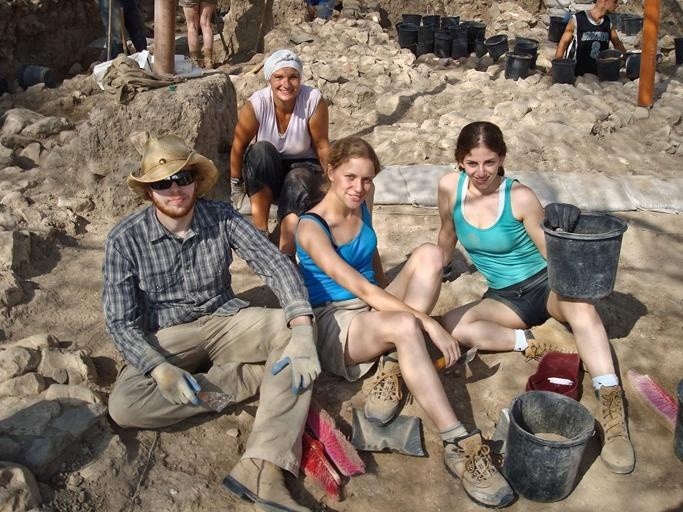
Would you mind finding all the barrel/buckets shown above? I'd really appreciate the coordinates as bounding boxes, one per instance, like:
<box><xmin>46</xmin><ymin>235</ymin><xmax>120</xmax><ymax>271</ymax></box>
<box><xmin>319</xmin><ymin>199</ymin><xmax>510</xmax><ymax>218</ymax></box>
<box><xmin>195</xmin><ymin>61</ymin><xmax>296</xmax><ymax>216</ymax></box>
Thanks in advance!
<box><xmin>513</xmin><ymin>38</ymin><xmax>539</xmax><ymax>69</ymax></box>
<box><xmin>548</xmin><ymin>16</ymin><xmax>568</xmax><ymax>43</ymax></box>
<box><xmin>606</xmin><ymin>12</ymin><xmax>643</xmax><ymax>36</ymax></box>
<box><xmin>504</xmin><ymin>52</ymin><xmax>533</xmax><ymax>81</ymax></box>
<box><xmin>551</xmin><ymin>59</ymin><xmax>576</xmax><ymax>83</ymax></box>
<box><xmin>673</xmin><ymin>38</ymin><xmax>683</xmax><ymax>65</ymax></box>
<box><xmin>621</xmin><ymin>51</ymin><xmax>641</xmax><ymax>81</ymax></box>
<box><xmin>502</xmin><ymin>391</ymin><xmax>596</xmax><ymax>503</ymax></box>
<box><xmin>16</xmin><ymin>63</ymin><xmax>57</xmax><ymax>88</ymax></box>
<box><xmin>483</xmin><ymin>34</ymin><xmax>509</xmax><ymax>61</ymax></box>
<box><xmin>540</xmin><ymin>211</ymin><xmax>628</xmax><ymax>300</ymax></box>
<box><xmin>395</xmin><ymin>11</ymin><xmax>486</xmax><ymax>59</ymax></box>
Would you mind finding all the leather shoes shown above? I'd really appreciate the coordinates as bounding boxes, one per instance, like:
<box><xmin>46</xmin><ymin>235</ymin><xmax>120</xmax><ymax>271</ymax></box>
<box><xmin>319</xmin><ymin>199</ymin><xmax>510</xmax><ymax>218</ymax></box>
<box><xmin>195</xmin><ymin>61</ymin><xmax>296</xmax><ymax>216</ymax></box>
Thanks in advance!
<box><xmin>221</xmin><ymin>457</ymin><xmax>313</xmax><ymax>511</ymax></box>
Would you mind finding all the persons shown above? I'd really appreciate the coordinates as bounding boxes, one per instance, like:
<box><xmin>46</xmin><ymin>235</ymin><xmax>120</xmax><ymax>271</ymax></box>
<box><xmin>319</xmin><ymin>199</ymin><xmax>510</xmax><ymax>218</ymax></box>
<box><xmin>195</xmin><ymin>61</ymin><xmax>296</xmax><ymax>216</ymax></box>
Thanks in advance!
<box><xmin>102</xmin><ymin>136</ymin><xmax>320</xmax><ymax>512</ymax></box>
<box><xmin>177</xmin><ymin>0</ymin><xmax>217</xmax><ymax>70</ymax></box>
<box><xmin>229</xmin><ymin>49</ymin><xmax>332</xmax><ymax>259</ymax></box>
<box><xmin>555</xmin><ymin>0</ymin><xmax>625</xmax><ymax>76</ymax></box>
<box><xmin>438</xmin><ymin>124</ymin><xmax>635</xmax><ymax>474</ymax></box>
<box><xmin>292</xmin><ymin>136</ymin><xmax>516</xmax><ymax>508</ymax></box>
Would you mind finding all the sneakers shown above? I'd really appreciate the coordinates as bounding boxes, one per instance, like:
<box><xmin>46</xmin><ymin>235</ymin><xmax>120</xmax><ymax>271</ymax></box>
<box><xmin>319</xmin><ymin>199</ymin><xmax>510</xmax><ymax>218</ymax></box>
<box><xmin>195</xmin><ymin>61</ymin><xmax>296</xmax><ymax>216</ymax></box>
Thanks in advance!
<box><xmin>442</xmin><ymin>428</ymin><xmax>515</xmax><ymax>508</ymax></box>
<box><xmin>522</xmin><ymin>316</ymin><xmax>588</xmax><ymax>372</ymax></box>
<box><xmin>593</xmin><ymin>384</ymin><xmax>636</xmax><ymax>474</ymax></box>
<box><xmin>203</xmin><ymin>57</ymin><xmax>213</xmax><ymax>69</ymax></box>
<box><xmin>363</xmin><ymin>354</ymin><xmax>403</xmax><ymax>427</ymax></box>
<box><xmin>184</xmin><ymin>55</ymin><xmax>198</xmax><ymax>66</ymax></box>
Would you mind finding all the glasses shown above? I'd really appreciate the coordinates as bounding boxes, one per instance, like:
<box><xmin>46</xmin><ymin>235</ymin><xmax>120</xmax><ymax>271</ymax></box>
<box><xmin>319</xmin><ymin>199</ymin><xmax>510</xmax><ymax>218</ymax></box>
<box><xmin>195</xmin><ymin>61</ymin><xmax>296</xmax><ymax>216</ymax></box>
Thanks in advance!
<box><xmin>149</xmin><ymin>170</ymin><xmax>197</xmax><ymax>190</ymax></box>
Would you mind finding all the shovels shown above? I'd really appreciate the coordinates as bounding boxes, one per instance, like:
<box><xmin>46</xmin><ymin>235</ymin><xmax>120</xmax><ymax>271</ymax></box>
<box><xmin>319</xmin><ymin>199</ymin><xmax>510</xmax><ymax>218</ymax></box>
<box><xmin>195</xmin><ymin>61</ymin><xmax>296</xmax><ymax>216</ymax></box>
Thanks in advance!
<box><xmin>351</xmin><ymin>408</ymin><xmax>425</xmax><ymax>457</ymax></box>
<box><xmin>434</xmin><ymin>345</ymin><xmax>479</xmax><ymax>371</ymax></box>
<box><xmin>193</xmin><ymin>391</ymin><xmax>234</xmax><ymax>414</ymax></box>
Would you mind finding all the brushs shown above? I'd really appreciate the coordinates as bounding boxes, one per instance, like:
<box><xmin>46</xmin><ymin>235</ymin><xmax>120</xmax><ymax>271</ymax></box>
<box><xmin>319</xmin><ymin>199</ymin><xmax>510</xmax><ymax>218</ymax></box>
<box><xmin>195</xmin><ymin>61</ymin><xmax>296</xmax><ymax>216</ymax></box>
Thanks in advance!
<box><xmin>297</xmin><ymin>399</ymin><xmax>365</xmax><ymax>502</ymax></box>
<box><xmin>627</xmin><ymin>369</ymin><xmax>677</xmax><ymax>428</ymax></box>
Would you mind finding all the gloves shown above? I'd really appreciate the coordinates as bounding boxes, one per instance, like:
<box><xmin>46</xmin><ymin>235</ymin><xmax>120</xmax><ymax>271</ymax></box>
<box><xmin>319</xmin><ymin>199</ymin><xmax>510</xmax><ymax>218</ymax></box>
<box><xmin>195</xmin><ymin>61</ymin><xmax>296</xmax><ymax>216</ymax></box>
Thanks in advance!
<box><xmin>148</xmin><ymin>360</ymin><xmax>201</xmax><ymax>406</ymax></box>
<box><xmin>230</xmin><ymin>176</ymin><xmax>246</xmax><ymax>211</ymax></box>
<box><xmin>270</xmin><ymin>324</ymin><xmax>321</xmax><ymax>398</ymax></box>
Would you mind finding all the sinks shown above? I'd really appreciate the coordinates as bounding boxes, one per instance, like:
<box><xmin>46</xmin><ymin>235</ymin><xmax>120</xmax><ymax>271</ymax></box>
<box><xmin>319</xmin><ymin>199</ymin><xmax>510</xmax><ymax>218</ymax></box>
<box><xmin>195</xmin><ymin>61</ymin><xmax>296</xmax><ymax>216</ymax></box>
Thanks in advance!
<box><xmin>597</xmin><ymin>49</ymin><xmax>624</xmax><ymax>81</ymax></box>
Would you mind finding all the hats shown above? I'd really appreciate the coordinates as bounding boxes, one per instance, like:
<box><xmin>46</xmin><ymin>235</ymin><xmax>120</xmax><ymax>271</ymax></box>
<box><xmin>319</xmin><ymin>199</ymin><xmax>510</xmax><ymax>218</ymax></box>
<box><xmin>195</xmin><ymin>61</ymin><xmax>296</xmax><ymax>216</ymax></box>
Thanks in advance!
<box><xmin>524</xmin><ymin>350</ymin><xmax>582</xmax><ymax>401</ymax></box>
<box><xmin>126</xmin><ymin>134</ymin><xmax>219</xmax><ymax>201</ymax></box>
